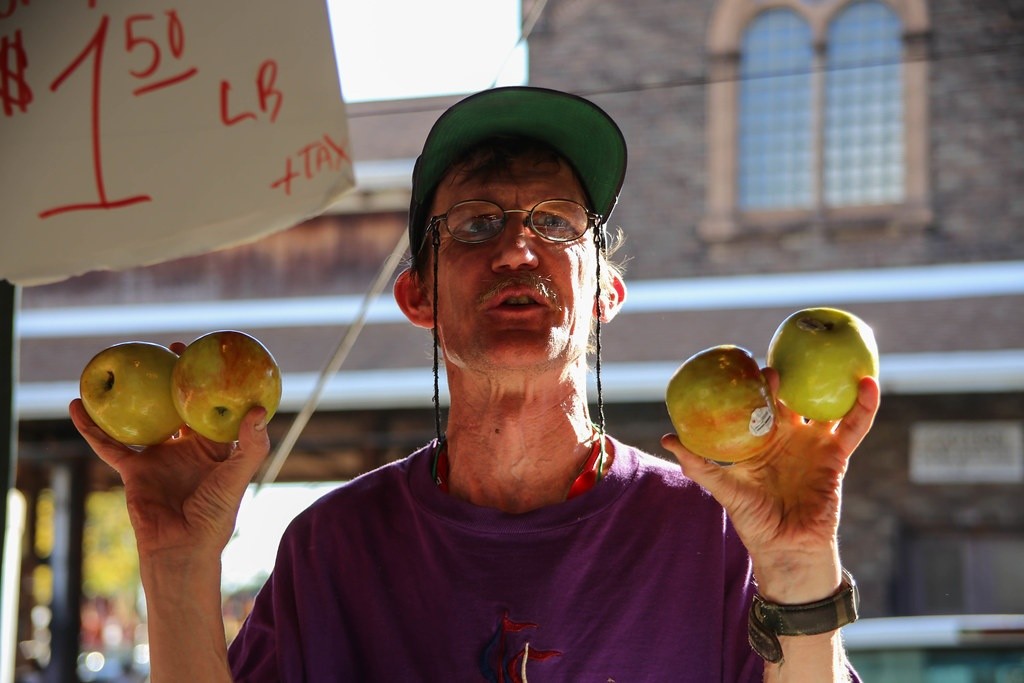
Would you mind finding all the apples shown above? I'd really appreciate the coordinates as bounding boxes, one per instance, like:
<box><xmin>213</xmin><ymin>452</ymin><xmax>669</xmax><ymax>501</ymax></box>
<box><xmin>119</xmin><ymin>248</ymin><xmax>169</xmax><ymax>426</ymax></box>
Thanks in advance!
<box><xmin>79</xmin><ymin>340</ymin><xmax>190</xmax><ymax>449</ymax></box>
<box><xmin>765</xmin><ymin>308</ymin><xmax>878</xmax><ymax>422</ymax></box>
<box><xmin>665</xmin><ymin>344</ymin><xmax>780</xmax><ymax>465</ymax></box>
<box><xmin>171</xmin><ymin>329</ymin><xmax>282</xmax><ymax>444</ymax></box>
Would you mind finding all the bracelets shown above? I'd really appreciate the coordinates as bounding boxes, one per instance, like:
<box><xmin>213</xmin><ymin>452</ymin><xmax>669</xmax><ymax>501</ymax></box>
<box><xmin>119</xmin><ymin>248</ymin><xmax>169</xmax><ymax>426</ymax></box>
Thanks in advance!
<box><xmin>746</xmin><ymin>573</ymin><xmax>858</xmax><ymax>662</ymax></box>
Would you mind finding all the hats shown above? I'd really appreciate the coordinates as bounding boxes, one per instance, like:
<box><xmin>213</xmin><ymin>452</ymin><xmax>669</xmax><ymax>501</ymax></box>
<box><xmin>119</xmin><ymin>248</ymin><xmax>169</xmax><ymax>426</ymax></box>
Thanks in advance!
<box><xmin>407</xmin><ymin>85</ymin><xmax>628</xmax><ymax>261</ymax></box>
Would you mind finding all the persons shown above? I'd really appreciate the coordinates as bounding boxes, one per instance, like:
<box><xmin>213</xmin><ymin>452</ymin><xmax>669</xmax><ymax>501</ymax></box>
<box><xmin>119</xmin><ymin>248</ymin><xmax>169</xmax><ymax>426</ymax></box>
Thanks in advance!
<box><xmin>69</xmin><ymin>86</ymin><xmax>882</xmax><ymax>683</ymax></box>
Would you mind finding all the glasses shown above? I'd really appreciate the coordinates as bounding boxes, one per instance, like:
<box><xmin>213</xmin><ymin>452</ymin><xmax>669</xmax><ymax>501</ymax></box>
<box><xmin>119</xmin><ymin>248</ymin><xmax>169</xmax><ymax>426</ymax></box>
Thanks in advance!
<box><xmin>415</xmin><ymin>199</ymin><xmax>607</xmax><ymax>256</ymax></box>
